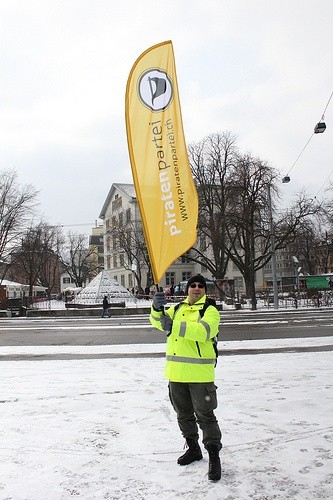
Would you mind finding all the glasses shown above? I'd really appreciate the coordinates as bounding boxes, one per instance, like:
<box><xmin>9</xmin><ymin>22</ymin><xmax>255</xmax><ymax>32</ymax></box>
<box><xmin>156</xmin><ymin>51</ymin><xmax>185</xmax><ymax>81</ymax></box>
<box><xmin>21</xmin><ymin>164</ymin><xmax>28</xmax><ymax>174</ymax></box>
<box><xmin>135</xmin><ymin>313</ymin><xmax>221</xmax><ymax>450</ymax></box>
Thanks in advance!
<box><xmin>190</xmin><ymin>284</ymin><xmax>204</xmax><ymax>288</ymax></box>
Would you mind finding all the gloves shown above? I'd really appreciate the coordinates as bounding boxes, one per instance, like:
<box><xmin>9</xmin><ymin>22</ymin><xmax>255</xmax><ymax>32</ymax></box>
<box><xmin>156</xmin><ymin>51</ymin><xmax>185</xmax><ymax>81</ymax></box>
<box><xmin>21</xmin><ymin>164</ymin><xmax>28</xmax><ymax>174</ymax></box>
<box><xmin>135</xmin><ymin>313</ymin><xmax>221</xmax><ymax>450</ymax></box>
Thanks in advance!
<box><xmin>159</xmin><ymin>312</ymin><xmax>172</xmax><ymax>331</ymax></box>
<box><xmin>153</xmin><ymin>292</ymin><xmax>167</xmax><ymax>309</ymax></box>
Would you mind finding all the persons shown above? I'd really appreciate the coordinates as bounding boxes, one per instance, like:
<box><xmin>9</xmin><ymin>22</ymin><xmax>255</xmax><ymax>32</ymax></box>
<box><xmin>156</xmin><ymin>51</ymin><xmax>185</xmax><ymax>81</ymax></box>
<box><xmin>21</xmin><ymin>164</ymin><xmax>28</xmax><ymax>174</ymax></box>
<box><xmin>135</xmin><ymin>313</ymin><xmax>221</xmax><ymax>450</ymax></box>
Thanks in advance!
<box><xmin>150</xmin><ymin>274</ymin><xmax>222</xmax><ymax>483</ymax></box>
<box><xmin>101</xmin><ymin>294</ymin><xmax>112</xmax><ymax>319</ymax></box>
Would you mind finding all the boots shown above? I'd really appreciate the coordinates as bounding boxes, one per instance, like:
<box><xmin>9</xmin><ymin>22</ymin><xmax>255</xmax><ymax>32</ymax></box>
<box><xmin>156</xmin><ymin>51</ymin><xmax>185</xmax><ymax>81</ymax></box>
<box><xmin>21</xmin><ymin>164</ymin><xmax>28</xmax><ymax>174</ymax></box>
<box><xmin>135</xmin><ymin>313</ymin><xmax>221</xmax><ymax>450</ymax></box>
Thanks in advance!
<box><xmin>206</xmin><ymin>448</ymin><xmax>221</xmax><ymax>480</ymax></box>
<box><xmin>177</xmin><ymin>439</ymin><xmax>203</xmax><ymax>466</ymax></box>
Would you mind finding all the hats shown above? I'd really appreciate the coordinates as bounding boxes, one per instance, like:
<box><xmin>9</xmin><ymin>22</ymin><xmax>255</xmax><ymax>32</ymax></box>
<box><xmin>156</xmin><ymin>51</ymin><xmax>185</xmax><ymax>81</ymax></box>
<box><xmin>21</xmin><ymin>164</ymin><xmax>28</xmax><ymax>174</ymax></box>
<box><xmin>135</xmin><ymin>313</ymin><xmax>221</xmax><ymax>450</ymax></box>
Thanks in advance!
<box><xmin>186</xmin><ymin>275</ymin><xmax>207</xmax><ymax>294</ymax></box>
